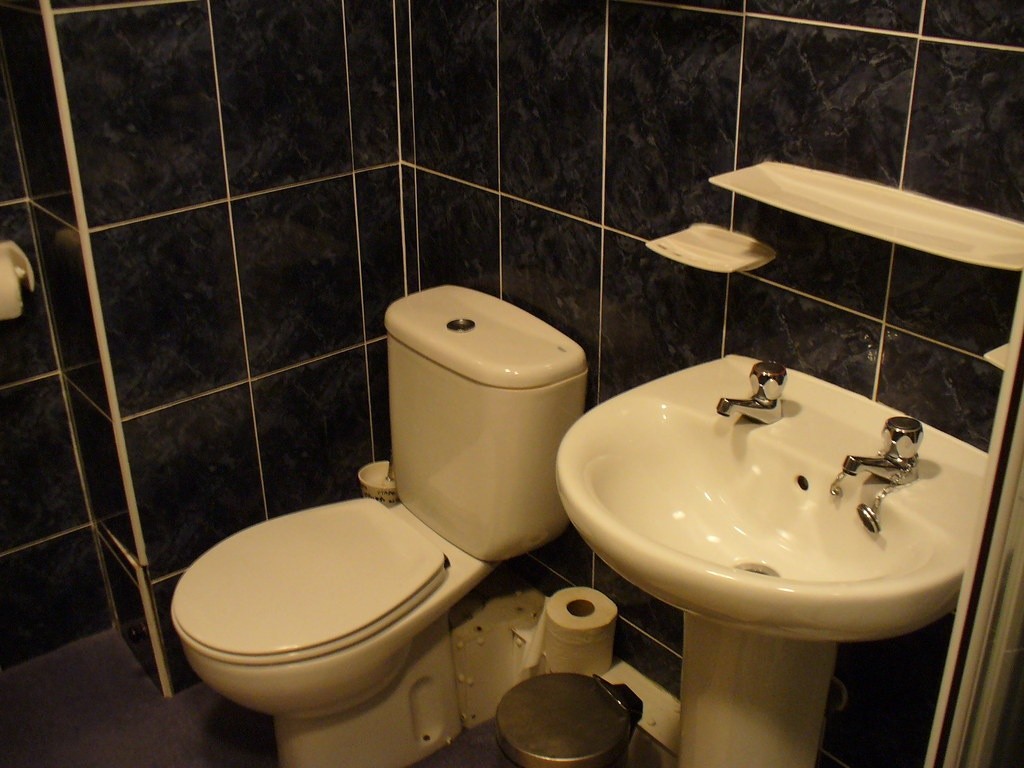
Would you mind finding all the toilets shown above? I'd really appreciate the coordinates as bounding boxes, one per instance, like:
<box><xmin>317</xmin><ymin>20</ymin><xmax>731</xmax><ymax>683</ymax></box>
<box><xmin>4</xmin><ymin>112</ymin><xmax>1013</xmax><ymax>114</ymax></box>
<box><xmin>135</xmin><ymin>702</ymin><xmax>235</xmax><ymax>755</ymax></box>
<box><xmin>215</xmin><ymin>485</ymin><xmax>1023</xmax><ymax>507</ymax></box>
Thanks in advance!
<box><xmin>168</xmin><ymin>282</ymin><xmax>592</xmax><ymax>768</ymax></box>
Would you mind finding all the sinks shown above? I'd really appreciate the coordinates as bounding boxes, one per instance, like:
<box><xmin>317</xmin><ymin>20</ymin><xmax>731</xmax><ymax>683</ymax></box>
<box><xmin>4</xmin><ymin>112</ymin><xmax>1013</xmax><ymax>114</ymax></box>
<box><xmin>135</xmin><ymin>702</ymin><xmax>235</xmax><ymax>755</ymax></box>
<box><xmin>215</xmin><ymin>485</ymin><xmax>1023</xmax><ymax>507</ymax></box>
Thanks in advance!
<box><xmin>552</xmin><ymin>352</ymin><xmax>990</xmax><ymax>645</ymax></box>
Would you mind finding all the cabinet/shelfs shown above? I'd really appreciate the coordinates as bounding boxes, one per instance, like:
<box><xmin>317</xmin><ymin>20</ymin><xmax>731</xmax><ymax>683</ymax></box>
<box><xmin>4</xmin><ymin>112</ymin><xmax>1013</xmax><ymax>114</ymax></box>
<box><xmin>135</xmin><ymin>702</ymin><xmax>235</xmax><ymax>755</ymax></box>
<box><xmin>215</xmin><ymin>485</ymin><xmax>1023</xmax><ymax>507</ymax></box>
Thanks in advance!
<box><xmin>645</xmin><ymin>162</ymin><xmax>1024</xmax><ymax>371</ymax></box>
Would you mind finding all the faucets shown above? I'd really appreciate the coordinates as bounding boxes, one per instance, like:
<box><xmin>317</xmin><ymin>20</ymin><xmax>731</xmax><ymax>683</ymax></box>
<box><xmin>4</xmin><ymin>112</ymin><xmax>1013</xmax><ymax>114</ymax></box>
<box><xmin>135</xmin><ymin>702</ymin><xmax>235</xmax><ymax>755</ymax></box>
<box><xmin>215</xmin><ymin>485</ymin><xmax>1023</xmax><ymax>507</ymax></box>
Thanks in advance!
<box><xmin>712</xmin><ymin>357</ymin><xmax>790</xmax><ymax>426</ymax></box>
<box><xmin>842</xmin><ymin>413</ymin><xmax>923</xmax><ymax>489</ymax></box>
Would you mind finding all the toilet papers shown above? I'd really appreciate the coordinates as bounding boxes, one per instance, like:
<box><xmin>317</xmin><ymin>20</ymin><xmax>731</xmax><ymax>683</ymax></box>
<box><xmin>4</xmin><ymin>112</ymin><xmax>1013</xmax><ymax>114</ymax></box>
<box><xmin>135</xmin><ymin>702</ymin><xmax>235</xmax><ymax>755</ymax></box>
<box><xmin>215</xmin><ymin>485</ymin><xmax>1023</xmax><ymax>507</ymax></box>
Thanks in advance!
<box><xmin>0</xmin><ymin>238</ymin><xmax>30</xmax><ymax>322</ymax></box>
<box><xmin>525</xmin><ymin>584</ymin><xmax>619</xmax><ymax>678</ymax></box>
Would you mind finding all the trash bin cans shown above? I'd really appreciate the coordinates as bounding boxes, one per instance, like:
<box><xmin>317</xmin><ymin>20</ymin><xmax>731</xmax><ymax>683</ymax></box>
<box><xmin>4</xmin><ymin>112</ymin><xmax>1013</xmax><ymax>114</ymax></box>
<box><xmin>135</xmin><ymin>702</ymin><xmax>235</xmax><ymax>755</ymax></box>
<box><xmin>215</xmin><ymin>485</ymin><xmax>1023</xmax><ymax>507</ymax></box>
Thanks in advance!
<box><xmin>495</xmin><ymin>672</ymin><xmax>642</xmax><ymax>768</ymax></box>
